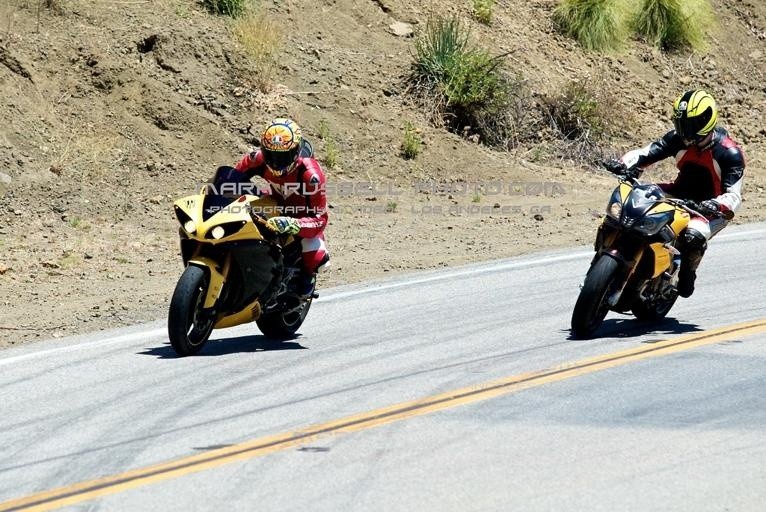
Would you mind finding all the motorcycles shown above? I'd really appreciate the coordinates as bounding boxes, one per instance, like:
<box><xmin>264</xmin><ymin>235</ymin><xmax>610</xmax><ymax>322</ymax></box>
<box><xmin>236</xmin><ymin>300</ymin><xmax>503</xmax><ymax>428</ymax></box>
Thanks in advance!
<box><xmin>168</xmin><ymin>165</ymin><xmax>318</xmax><ymax>356</ymax></box>
<box><xmin>571</xmin><ymin>170</ymin><xmax>728</xmax><ymax>340</ymax></box>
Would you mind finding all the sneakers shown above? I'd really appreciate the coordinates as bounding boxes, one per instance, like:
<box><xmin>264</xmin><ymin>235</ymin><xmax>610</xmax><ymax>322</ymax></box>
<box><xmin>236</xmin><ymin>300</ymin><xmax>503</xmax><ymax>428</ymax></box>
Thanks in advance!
<box><xmin>295</xmin><ymin>271</ymin><xmax>316</xmax><ymax>301</ymax></box>
<box><xmin>676</xmin><ymin>270</ymin><xmax>695</xmax><ymax>298</ymax></box>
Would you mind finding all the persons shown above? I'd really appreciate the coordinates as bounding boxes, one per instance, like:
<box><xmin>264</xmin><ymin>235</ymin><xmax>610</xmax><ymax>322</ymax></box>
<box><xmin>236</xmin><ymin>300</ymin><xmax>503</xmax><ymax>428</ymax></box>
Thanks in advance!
<box><xmin>234</xmin><ymin>118</ymin><xmax>329</xmax><ymax>316</ymax></box>
<box><xmin>603</xmin><ymin>89</ymin><xmax>746</xmax><ymax>298</ymax></box>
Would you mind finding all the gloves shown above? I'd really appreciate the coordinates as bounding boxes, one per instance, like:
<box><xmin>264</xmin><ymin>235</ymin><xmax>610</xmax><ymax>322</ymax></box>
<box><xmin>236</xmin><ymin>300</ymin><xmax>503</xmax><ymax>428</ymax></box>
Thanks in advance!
<box><xmin>699</xmin><ymin>199</ymin><xmax>721</xmax><ymax>216</ymax></box>
<box><xmin>266</xmin><ymin>217</ymin><xmax>301</xmax><ymax>235</ymax></box>
<box><xmin>607</xmin><ymin>158</ymin><xmax>627</xmax><ymax>175</ymax></box>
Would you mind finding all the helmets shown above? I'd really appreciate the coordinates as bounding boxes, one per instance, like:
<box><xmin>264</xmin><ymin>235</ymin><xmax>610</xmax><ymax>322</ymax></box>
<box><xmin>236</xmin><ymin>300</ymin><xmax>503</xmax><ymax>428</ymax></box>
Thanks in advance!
<box><xmin>671</xmin><ymin>88</ymin><xmax>717</xmax><ymax>149</ymax></box>
<box><xmin>262</xmin><ymin>118</ymin><xmax>303</xmax><ymax>177</ymax></box>
